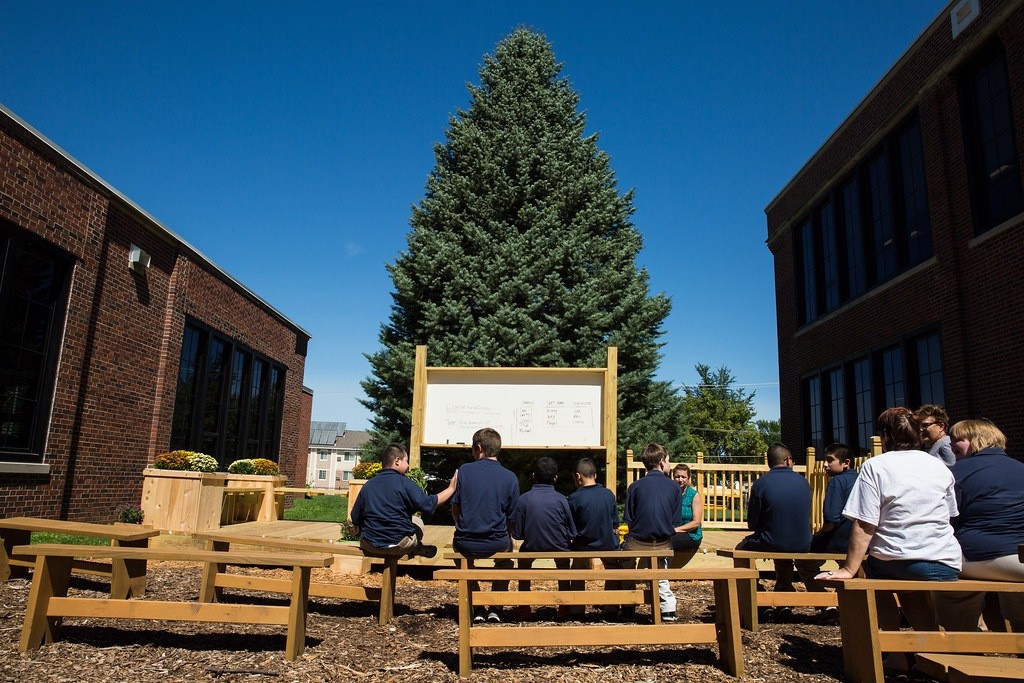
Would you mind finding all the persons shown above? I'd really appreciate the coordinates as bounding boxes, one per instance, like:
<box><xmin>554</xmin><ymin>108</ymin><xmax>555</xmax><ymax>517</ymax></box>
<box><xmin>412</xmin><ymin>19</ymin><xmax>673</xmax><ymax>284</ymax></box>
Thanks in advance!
<box><xmin>795</xmin><ymin>444</ymin><xmax>859</xmax><ymax>619</ymax></box>
<box><xmin>736</xmin><ymin>442</ymin><xmax>813</xmax><ymax>618</ymax></box>
<box><xmin>671</xmin><ymin>463</ymin><xmax>702</xmax><ymax>551</ymax></box>
<box><xmin>618</xmin><ymin>443</ymin><xmax>679</xmax><ymax>621</ymax></box>
<box><xmin>813</xmin><ymin>405</ymin><xmax>1024</xmax><ymax>678</ymax></box>
<box><xmin>512</xmin><ymin>457</ymin><xmax>578</xmax><ymax>617</ymax></box>
<box><xmin>567</xmin><ymin>458</ymin><xmax>621</xmax><ymax>616</ymax></box>
<box><xmin>450</xmin><ymin>428</ymin><xmax>520</xmax><ymax>621</ymax></box>
<box><xmin>349</xmin><ymin>443</ymin><xmax>458</xmax><ymax>555</ymax></box>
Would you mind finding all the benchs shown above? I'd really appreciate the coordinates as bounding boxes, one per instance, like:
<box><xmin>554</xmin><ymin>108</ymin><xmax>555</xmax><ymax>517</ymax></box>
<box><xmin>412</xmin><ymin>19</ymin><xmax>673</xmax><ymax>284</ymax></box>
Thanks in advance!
<box><xmin>276</xmin><ymin>488</ymin><xmax>349</xmax><ymax>494</ymax></box>
<box><xmin>1</xmin><ymin>516</ymin><xmax>1024</xmax><ymax>683</ymax></box>
<box><xmin>220</xmin><ymin>487</ymin><xmax>264</xmax><ymax>525</ymax></box>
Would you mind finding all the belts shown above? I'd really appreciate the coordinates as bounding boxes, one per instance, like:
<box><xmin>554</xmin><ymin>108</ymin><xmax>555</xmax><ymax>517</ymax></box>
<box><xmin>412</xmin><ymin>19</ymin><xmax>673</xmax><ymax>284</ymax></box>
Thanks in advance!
<box><xmin>632</xmin><ymin>536</ymin><xmax>668</xmax><ymax>543</ymax></box>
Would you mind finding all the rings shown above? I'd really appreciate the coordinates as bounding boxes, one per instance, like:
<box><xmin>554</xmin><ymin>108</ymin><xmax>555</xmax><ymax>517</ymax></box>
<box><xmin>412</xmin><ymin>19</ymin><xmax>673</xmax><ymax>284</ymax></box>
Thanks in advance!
<box><xmin>828</xmin><ymin>572</ymin><xmax>833</xmax><ymax>575</ymax></box>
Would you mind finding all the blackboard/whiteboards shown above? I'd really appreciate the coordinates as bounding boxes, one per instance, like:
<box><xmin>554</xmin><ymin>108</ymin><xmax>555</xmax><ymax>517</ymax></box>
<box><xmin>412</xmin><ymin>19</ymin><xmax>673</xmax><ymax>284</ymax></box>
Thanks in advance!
<box><xmin>424</xmin><ymin>384</ymin><xmax>601</xmax><ymax>447</ymax></box>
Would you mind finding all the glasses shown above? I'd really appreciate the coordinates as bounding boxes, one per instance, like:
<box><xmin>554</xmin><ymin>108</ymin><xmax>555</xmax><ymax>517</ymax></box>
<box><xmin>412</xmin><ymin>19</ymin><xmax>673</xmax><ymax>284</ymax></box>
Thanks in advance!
<box><xmin>916</xmin><ymin>422</ymin><xmax>938</xmax><ymax>431</ymax></box>
<box><xmin>784</xmin><ymin>457</ymin><xmax>793</xmax><ymax>463</ymax></box>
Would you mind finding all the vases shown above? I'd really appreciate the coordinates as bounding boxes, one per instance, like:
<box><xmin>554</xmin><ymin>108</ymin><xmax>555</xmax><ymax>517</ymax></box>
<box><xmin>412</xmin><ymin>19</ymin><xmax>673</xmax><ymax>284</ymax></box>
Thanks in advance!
<box><xmin>142</xmin><ymin>469</ymin><xmax>227</xmax><ymax>533</ymax></box>
<box><xmin>229</xmin><ymin>476</ymin><xmax>286</xmax><ymax>520</ymax></box>
<box><xmin>349</xmin><ymin>479</ymin><xmax>367</xmax><ymax>517</ymax></box>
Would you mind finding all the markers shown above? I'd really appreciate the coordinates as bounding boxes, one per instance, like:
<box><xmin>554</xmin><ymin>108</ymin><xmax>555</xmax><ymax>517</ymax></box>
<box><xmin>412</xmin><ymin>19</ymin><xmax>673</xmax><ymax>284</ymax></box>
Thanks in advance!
<box><xmin>457</xmin><ymin>442</ymin><xmax>464</xmax><ymax>444</ymax></box>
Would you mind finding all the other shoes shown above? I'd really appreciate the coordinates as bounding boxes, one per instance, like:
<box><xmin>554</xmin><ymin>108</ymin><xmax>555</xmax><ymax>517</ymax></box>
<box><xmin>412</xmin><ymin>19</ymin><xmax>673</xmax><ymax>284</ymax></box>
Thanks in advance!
<box><xmin>758</xmin><ymin>607</ymin><xmax>773</xmax><ymax>623</ymax></box>
<box><xmin>910</xmin><ymin>663</ymin><xmax>939</xmax><ymax>682</ymax></box>
<box><xmin>557</xmin><ymin>607</ymin><xmax>568</xmax><ymax>616</ymax></box>
<box><xmin>474</xmin><ymin>608</ymin><xmax>487</xmax><ymax>622</ymax></box>
<box><xmin>488</xmin><ymin>606</ymin><xmax>501</xmax><ymax>622</ymax></box>
<box><xmin>621</xmin><ymin>609</ymin><xmax>636</xmax><ymax>621</ymax></box>
<box><xmin>512</xmin><ymin>605</ymin><xmax>531</xmax><ymax>617</ymax></box>
<box><xmin>819</xmin><ymin>607</ymin><xmax>839</xmax><ymax>624</ymax></box>
<box><xmin>661</xmin><ymin>612</ymin><xmax>675</xmax><ymax>621</ymax></box>
<box><xmin>883</xmin><ymin>659</ymin><xmax>907</xmax><ymax>678</ymax></box>
<box><xmin>776</xmin><ymin>606</ymin><xmax>792</xmax><ymax>623</ymax></box>
<box><xmin>570</xmin><ymin>605</ymin><xmax>586</xmax><ymax>616</ymax></box>
<box><xmin>601</xmin><ymin>608</ymin><xmax>618</xmax><ymax>618</ymax></box>
<box><xmin>411</xmin><ymin>544</ymin><xmax>437</xmax><ymax>558</ymax></box>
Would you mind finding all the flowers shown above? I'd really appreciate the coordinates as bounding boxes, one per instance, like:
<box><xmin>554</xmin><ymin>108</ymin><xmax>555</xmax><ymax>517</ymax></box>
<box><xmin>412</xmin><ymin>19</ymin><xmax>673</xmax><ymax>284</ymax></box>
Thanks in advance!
<box><xmin>157</xmin><ymin>450</ymin><xmax>280</xmax><ymax>475</ymax></box>
<box><xmin>351</xmin><ymin>461</ymin><xmax>383</xmax><ymax>477</ymax></box>
<box><xmin>118</xmin><ymin>507</ymin><xmax>143</xmax><ymax>522</ymax></box>
